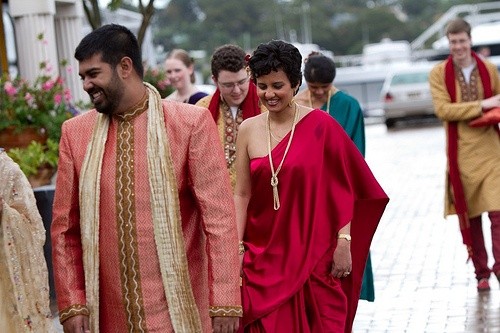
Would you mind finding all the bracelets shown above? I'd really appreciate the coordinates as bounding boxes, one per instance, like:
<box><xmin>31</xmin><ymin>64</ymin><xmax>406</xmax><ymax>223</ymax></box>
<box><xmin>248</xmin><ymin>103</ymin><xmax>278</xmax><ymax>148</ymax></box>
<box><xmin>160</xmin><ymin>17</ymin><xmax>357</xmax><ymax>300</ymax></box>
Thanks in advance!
<box><xmin>239</xmin><ymin>241</ymin><xmax>245</xmax><ymax>255</ymax></box>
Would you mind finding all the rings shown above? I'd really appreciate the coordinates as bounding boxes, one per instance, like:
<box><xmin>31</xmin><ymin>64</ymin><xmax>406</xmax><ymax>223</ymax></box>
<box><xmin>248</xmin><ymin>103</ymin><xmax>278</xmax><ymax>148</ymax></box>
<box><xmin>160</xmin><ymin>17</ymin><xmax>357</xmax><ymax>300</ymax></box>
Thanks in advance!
<box><xmin>344</xmin><ymin>272</ymin><xmax>350</xmax><ymax>275</ymax></box>
<box><xmin>338</xmin><ymin>271</ymin><xmax>343</xmax><ymax>276</ymax></box>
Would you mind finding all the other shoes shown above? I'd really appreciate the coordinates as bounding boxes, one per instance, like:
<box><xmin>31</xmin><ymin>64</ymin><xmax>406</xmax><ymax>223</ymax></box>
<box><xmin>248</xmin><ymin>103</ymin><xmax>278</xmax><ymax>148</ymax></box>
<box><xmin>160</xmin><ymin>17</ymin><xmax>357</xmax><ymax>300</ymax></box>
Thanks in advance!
<box><xmin>477</xmin><ymin>277</ymin><xmax>490</xmax><ymax>290</ymax></box>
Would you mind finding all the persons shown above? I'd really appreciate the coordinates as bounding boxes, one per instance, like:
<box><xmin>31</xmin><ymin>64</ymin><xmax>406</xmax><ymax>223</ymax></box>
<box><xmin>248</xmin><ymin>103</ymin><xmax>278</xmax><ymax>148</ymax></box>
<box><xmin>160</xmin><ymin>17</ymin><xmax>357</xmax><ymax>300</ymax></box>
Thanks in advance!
<box><xmin>0</xmin><ymin>23</ymin><xmax>389</xmax><ymax>333</ymax></box>
<box><xmin>428</xmin><ymin>20</ymin><xmax>500</xmax><ymax>291</ymax></box>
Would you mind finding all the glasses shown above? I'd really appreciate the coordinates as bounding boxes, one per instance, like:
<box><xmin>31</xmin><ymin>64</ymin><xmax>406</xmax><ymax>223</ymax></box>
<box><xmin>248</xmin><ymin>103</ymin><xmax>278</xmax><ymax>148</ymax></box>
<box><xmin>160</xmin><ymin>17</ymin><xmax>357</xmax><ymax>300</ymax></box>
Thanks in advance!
<box><xmin>217</xmin><ymin>70</ymin><xmax>249</xmax><ymax>89</ymax></box>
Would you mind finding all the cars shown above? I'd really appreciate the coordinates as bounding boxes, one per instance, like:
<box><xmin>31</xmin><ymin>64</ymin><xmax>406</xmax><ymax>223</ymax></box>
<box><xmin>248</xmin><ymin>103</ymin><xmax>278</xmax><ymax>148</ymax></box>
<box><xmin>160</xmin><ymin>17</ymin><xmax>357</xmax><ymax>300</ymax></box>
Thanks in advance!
<box><xmin>380</xmin><ymin>64</ymin><xmax>452</xmax><ymax>129</ymax></box>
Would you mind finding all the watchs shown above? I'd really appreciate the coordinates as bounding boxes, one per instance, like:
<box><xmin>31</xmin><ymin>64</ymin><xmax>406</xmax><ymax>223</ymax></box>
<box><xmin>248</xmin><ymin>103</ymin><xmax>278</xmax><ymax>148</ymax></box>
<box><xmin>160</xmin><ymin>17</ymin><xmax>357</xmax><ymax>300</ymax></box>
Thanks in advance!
<box><xmin>336</xmin><ymin>234</ymin><xmax>351</xmax><ymax>242</ymax></box>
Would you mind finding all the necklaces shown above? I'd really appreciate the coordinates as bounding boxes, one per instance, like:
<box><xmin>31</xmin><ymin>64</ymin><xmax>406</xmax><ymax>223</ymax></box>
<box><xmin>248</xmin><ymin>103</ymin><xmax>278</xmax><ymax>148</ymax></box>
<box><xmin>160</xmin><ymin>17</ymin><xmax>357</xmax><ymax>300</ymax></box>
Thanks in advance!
<box><xmin>265</xmin><ymin>102</ymin><xmax>301</xmax><ymax>211</ymax></box>
<box><xmin>308</xmin><ymin>89</ymin><xmax>331</xmax><ymax>117</ymax></box>
